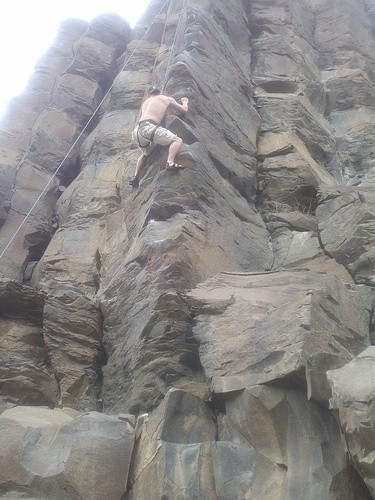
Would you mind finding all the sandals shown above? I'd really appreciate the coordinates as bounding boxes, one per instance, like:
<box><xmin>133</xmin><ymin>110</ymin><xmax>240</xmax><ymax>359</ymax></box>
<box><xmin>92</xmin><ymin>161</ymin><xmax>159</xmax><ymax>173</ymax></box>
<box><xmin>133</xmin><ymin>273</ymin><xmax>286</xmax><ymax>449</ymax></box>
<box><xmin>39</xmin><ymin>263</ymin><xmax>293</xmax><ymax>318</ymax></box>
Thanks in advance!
<box><xmin>129</xmin><ymin>177</ymin><xmax>140</xmax><ymax>186</ymax></box>
<box><xmin>166</xmin><ymin>161</ymin><xmax>186</xmax><ymax>172</ymax></box>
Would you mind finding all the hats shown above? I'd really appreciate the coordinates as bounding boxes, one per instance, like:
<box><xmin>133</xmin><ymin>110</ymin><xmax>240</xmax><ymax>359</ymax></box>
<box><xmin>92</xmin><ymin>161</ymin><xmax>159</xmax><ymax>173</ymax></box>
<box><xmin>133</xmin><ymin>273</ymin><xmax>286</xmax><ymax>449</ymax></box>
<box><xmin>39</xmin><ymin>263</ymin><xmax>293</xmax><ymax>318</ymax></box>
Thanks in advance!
<box><xmin>148</xmin><ymin>85</ymin><xmax>161</xmax><ymax>95</ymax></box>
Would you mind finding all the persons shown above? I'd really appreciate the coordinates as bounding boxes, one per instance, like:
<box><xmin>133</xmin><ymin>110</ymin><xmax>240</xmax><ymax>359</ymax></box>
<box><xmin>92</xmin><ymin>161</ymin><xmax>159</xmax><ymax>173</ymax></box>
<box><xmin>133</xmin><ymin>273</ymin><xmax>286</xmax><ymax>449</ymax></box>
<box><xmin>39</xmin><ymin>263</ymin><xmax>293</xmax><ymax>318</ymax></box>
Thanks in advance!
<box><xmin>129</xmin><ymin>85</ymin><xmax>189</xmax><ymax>186</ymax></box>
